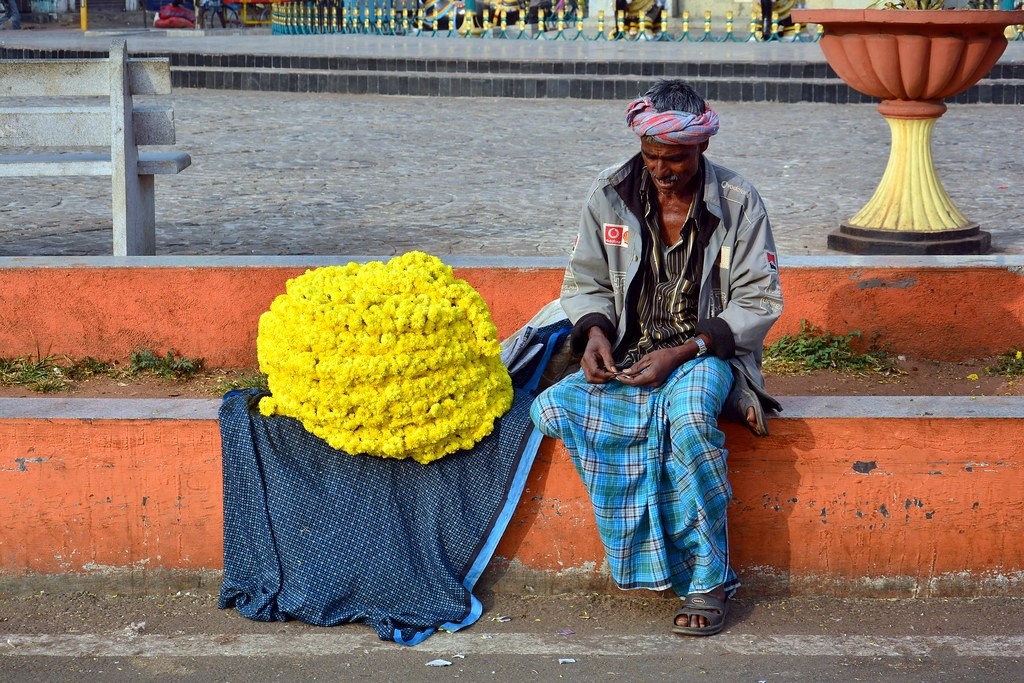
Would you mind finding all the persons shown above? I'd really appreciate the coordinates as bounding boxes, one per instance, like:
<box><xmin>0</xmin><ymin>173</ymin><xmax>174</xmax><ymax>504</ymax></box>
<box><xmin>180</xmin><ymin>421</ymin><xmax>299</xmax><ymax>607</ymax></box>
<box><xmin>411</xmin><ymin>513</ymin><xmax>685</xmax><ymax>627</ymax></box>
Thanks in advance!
<box><xmin>531</xmin><ymin>79</ymin><xmax>784</xmax><ymax>634</ymax></box>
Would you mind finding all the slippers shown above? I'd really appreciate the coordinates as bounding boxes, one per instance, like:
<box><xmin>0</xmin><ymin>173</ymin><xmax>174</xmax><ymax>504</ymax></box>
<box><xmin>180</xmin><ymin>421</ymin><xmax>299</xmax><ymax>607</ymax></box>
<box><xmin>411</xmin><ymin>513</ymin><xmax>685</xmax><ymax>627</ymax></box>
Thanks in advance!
<box><xmin>671</xmin><ymin>593</ymin><xmax>729</xmax><ymax>634</ymax></box>
<box><xmin>723</xmin><ymin>363</ymin><xmax>766</xmax><ymax>436</ymax></box>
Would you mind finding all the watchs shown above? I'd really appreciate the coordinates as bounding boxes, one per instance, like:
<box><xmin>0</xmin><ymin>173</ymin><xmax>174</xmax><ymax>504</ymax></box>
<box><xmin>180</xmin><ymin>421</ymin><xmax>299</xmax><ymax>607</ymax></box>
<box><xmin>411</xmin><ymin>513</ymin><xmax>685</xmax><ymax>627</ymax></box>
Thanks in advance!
<box><xmin>684</xmin><ymin>337</ymin><xmax>707</xmax><ymax>357</ymax></box>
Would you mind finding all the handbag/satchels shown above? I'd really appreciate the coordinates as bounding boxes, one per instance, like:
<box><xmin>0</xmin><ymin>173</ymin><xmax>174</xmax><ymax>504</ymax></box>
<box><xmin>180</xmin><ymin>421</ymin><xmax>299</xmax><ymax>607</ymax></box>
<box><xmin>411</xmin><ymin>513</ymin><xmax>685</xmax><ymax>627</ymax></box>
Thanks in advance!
<box><xmin>538</xmin><ymin>334</ymin><xmax>584</xmax><ymax>392</ymax></box>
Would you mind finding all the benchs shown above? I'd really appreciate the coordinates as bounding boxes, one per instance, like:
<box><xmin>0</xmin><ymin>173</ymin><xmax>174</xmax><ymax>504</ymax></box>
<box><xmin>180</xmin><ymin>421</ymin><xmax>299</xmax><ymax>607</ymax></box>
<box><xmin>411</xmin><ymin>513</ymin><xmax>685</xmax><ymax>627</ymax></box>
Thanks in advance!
<box><xmin>0</xmin><ymin>41</ymin><xmax>192</xmax><ymax>256</ymax></box>
